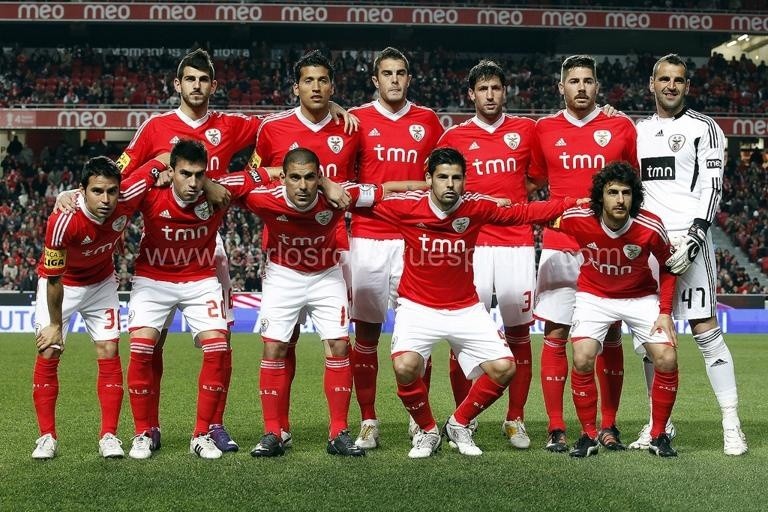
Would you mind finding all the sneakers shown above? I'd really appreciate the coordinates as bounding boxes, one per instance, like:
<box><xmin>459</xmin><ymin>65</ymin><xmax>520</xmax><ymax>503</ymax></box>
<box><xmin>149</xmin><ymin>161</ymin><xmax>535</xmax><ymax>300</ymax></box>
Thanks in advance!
<box><xmin>97</xmin><ymin>433</ymin><xmax>125</xmax><ymax>460</ymax></box>
<box><xmin>353</xmin><ymin>418</ymin><xmax>382</xmax><ymax>451</ymax></box>
<box><xmin>31</xmin><ymin>436</ymin><xmax>59</xmax><ymax>461</ymax></box>
<box><xmin>281</xmin><ymin>430</ymin><xmax>293</xmax><ymax>447</ymax></box>
<box><xmin>723</xmin><ymin>414</ymin><xmax>750</xmax><ymax>455</ymax></box>
<box><xmin>447</xmin><ymin>417</ymin><xmax>478</xmax><ymax>448</ymax></box>
<box><xmin>501</xmin><ymin>419</ymin><xmax>533</xmax><ymax>450</ymax></box>
<box><xmin>209</xmin><ymin>424</ymin><xmax>238</xmax><ymax>453</ymax></box>
<box><xmin>128</xmin><ymin>436</ymin><xmax>152</xmax><ymax>461</ymax></box>
<box><xmin>444</xmin><ymin>416</ymin><xmax>484</xmax><ymax>458</ymax></box>
<box><xmin>630</xmin><ymin>420</ymin><xmax>677</xmax><ymax>449</ymax></box>
<box><xmin>545</xmin><ymin>433</ymin><xmax>568</xmax><ymax>453</ymax></box>
<box><xmin>409</xmin><ymin>426</ymin><xmax>442</xmax><ymax>458</ymax></box>
<box><xmin>648</xmin><ymin>435</ymin><xmax>677</xmax><ymax>458</ymax></box>
<box><xmin>568</xmin><ymin>434</ymin><xmax>600</xmax><ymax>458</ymax></box>
<box><xmin>147</xmin><ymin>429</ymin><xmax>161</xmax><ymax>451</ymax></box>
<box><xmin>189</xmin><ymin>436</ymin><xmax>224</xmax><ymax>459</ymax></box>
<box><xmin>597</xmin><ymin>428</ymin><xmax>626</xmax><ymax>451</ymax></box>
<box><xmin>249</xmin><ymin>435</ymin><xmax>285</xmax><ymax>457</ymax></box>
<box><xmin>408</xmin><ymin>412</ymin><xmax>424</xmax><ymax>436</ymax></box>
<box><xmin>327</xmin><ymin>431</ymin><xmax>366</xmax><ymax>457</ymax></box>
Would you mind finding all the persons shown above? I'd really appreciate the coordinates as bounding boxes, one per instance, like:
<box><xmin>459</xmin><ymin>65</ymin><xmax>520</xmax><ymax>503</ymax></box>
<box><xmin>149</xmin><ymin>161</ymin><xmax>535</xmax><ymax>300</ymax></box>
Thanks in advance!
<box><xmin>0</xmin><ymin>192</ymin><xmax>55</xmax><ymax>292</ymax></box>
<box><xmin>153</xmin><ymin>148</ymin><xmax>462</xmax><ymax>457</ymax></box>
<box><xmin>685</xmin><ymin>52</ymin><xmax>768</xmax><ymax>116</ymax></box>
<box><xmin>500</xmin><ymin>161</ymin><xmax>680</xmax><ymax>459</ymax></box>
<box><xmin>714</xmin><ymin>137</ymin><xmax>768</xmax><ymax>297</ymax></box>
<box><xmin>218</xmin><ymin>144</ymin><xmax>267</xmax><ymax>296</ymax></box>
<box><xmin>0</xmin><ymin>41</ymin><xmax>181</xmax><ymax>110</ymax></box>
<box><xmin>0</xmin><ymin>160</ymin><xmax>87</xmax><ymax>192</ymax></box>
<box><xmin>629</xmin><ymin>53</ymin><xmax>749</xmax><ymax>457</ymax></box>
<box><xmin>523</xmin><ymin>55</ymin><xmax>642</xmax><ymax>452</ymax></box>
<box><xmin>112</xmin><ymin>48</ymin><xmax>362</xmax><ymax>455</ymax></box>
<box><xmin>1</xmin><ymin>132</ymin><xmax>129</xmax><ymax>160</ymax></box>
<box><xmin>331</xmin><ymin>48</ymin><xmax>378</xmax><ymax>106</ymax></box>
<box><xmin>53</xmin><ymin>139</ymin><xmax>352</xmax><ymax>460</ymax></box>
<box><xmin>210</xmin><ymin>44</ymin><xmax>300</xmax><ymax>109</ymax></box>
<box><xmin>31</xmin><ymin>155</ymin><xmax>232</xmax><ymax>460</ymax></box>
<box><xmin>346</xmin><ymin>47</ymin><xmax>446</xmax><ymax>456</ymax></box>
<box><xmin>527</xmin><ymin>184</ymin><xmax>550</xmax><ymax>278</ymax></box>
<box><xmin>593</xmin><ymin>54</ymin><xmax>656</xmax><ymax>113</ymax></box>
<box><xmin>354</xmin><ymin>149</ymin><xmax>592</xmax><ymax>457</ymax></box>
<box><xmin>109</xmin><ymin>210</ymin><xmax>145</xmax><ymax>292</ymax></box>
<box><xmin>426</xmin><ymin>59</ymin><xmax>544</xmax><ymax>449</ymax></box>
<box><xmin>243</xmin><ymin>49</ymin><xmax>366</xmax><ymax>450</ymax></box>
<box><xmin>405</xmin><ymin>49</ymin><xmax>476</xmax><ymax>114</ymax></box>
<box><xmin>499</xmin><ymin>58</ymin><xmax>565</xmax><ymax>114</ymax></box>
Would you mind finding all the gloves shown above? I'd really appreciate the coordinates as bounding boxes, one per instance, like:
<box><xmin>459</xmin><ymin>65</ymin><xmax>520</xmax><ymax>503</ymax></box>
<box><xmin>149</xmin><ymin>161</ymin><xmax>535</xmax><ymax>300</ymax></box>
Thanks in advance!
<box><xmin>665</xmin><ymin>219</ymin><xmax>713</xmax><ymax>276</ymax></box>
